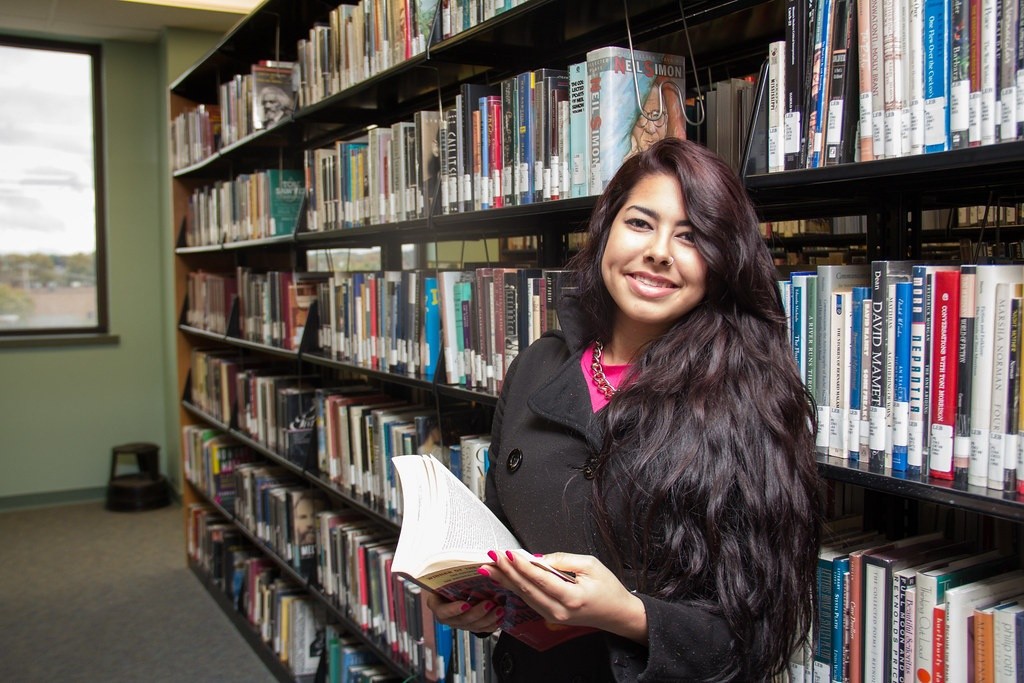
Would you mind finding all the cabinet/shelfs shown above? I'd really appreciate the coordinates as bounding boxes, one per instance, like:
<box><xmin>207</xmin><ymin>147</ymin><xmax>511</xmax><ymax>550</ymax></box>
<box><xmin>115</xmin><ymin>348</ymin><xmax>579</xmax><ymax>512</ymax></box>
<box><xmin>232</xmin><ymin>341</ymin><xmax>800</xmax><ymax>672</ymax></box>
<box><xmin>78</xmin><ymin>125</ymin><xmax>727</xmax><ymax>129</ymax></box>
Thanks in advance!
<box><xmin>156</xmin><ymin>2</ymin><xmax>1024</xmax><ymax>683</ymax></box>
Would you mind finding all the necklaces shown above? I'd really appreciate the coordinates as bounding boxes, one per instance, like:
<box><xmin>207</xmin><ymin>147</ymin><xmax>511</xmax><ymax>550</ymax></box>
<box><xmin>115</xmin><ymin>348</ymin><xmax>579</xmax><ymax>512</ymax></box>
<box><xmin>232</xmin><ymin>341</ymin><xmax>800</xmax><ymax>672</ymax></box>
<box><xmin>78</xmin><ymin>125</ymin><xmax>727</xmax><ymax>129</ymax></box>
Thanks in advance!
<box><xmin>591</xmin><ymin>327</ymin><xmax>626</xmax><ymax>401</ymax></box>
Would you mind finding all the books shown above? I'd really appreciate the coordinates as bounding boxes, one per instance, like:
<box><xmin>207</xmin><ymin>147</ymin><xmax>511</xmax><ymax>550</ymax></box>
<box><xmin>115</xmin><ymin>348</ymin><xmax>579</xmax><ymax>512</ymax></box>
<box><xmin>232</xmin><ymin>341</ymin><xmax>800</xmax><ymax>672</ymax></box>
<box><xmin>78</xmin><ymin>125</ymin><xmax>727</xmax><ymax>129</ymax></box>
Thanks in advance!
<box><xmin>425</xmin><ymin>170</ymin><xmax>603</xmax><ymax>392</ymax></box>
<box><xmin>170</xmin><ymin>1</ymin><xmax>424</xmax><ymax>680</ymax></box>
<box><xmin>385</xmin><ymin>454</ymin><xmax>603</xmax><ymax>654</ymax></box>
<box><xmin>432</xmin><ymin>1</ymin><xmax>1024</xmax><ymax>170</ymax></box>
<box><xmin>775</xmin><ymin>239</ymin><xmax>1024</xmax><ymax>681</ymax></box>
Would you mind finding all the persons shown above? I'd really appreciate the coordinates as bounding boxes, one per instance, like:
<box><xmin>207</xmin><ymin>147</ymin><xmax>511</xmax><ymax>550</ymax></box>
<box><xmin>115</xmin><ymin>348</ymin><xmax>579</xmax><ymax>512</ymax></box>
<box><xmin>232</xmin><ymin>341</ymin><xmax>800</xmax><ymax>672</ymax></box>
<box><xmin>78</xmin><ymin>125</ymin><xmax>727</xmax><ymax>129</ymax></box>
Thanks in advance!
<box><xmin>426</xmin><ymin>142</ymin><xmax>815</xmax><ymax>683</ymax></box>
